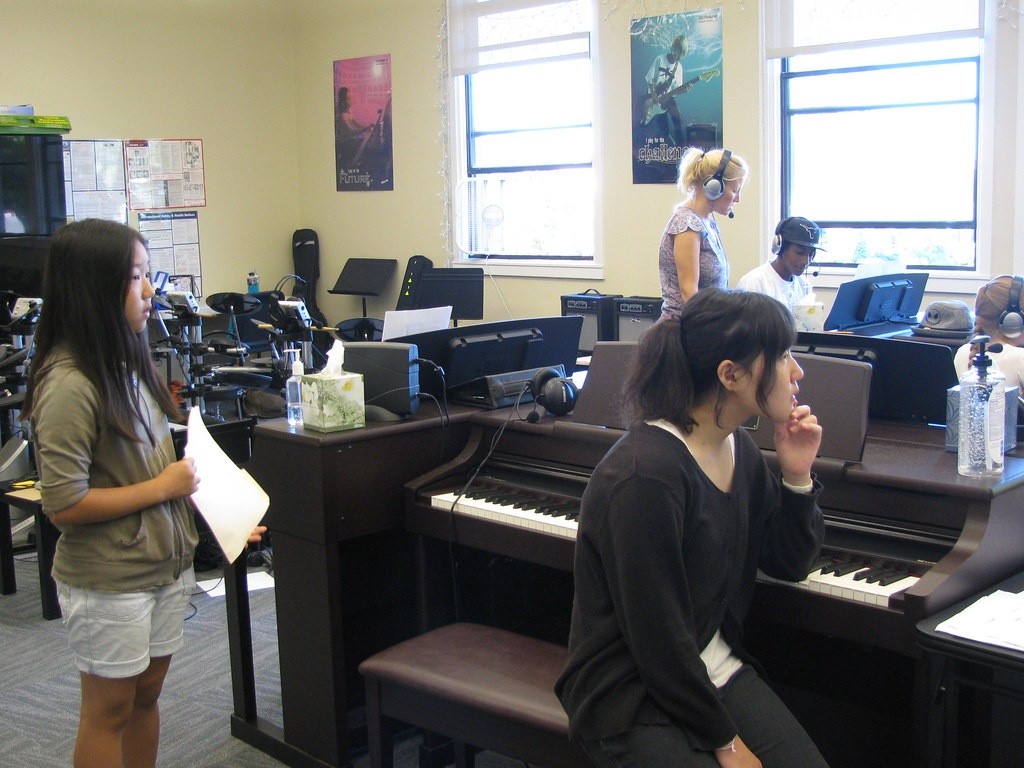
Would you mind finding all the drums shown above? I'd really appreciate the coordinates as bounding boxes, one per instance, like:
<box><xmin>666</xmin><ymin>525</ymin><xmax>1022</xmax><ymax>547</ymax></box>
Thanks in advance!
<box><xmin>202</xmin><ymin>330</ymin><xmax>239</xmax><ymax>347</ymax></box>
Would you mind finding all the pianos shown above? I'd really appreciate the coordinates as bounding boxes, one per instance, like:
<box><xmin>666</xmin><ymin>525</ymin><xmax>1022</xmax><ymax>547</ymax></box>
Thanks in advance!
<box><xmin>405</xmin><ymin>340</ymin><xmax>1024</xmax><ymax>768</ymax></box>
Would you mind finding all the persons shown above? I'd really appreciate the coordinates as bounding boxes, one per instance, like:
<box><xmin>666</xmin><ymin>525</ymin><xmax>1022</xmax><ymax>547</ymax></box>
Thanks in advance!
<box><xmin>553</xmin><ymin>288</ymin><xmax>828</xmax><ymax>768</ymax></box>
<box><xmin>659</xmin><ymin>147</ymin><xmax>749</xmax><ymax>318</ymax></box>
<box><xmin>337</xmin><ymin>87</ymin><xmax>376</xmax><ymax>153</ymax></box>
<box><xmin>17</xmin><ymin>218</ymin><xmax>267</xmax><ymax>768</ymax></box>
<box><xmin>954</xmin><ymin>274</ymin><xmax>1024</xmax><ymax>397</ymax></box>
<box><xmin>736</xmin><ymin>217</ymin><xmax>828</xmax><ymax>314</ymax></box>
<box><xmin>645</xmin><ymin>36</ymin><xmax>693</xmax><ymax>148</ymax></box>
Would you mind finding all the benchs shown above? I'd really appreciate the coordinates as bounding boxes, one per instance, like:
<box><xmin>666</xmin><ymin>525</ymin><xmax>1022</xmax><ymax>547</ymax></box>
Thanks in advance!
<box><xmin>359</xmin><ymin>622</ymin><xmax>584</xmax><ymax>767</ymax></box>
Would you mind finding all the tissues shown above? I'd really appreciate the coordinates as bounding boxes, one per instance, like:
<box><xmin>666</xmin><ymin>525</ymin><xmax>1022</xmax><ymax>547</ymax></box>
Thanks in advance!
<box><xmin>300</xmin><ymin>338</ymin><xmax>366</xmax><ymax>433</ymax></box>
<box><xmin>944</xmin><ymin>352</ymin><xmax>1020</xmax><ymax>454</ymax></box>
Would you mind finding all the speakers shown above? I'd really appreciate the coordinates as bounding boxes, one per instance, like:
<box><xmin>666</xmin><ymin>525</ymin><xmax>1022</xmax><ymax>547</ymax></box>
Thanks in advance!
<box><xmin>561</xmin><ymin>293</ymin><xmax>623</xmax><ymax>354</ymax></box>
<box><xmin>613</xmin><ymin>296</ymin><xmax>664</xmax><ymax>342</ymax></box>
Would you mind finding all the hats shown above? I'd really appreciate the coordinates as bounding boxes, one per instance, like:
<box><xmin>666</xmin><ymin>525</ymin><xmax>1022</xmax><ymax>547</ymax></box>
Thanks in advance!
<box><xmin>775</xmin><ymin>217</ymin><xmax>826</xmax><ymax>252</ymax></box>
<box><xmin>911</xmin><ymin>299</ymin><xmax>974</xmax><ymax>337</ymax></box>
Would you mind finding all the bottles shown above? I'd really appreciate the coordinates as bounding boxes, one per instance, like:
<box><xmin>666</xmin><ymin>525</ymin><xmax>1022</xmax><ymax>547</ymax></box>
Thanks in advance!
<box><xmin>246</xmin><ymin>272</ymin><xmax>260</xmax><ymax>293</ymax></box>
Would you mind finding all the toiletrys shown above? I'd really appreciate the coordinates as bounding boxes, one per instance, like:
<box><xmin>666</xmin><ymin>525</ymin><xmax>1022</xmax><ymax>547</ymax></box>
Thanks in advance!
<box><xmin>958</xmin><ymin>334</ymin><xmax>1007</xmax><ymax>478</ymax></box>
<box><xmin>283</xmin><ymin>348</ymin><xmax>304</xmax><ymax>426</ymax></box>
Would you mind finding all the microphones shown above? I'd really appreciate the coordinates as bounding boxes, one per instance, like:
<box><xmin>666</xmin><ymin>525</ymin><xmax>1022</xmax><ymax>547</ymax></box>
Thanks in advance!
<box><xmin>811</xmin><ymin>259</ymin><xmax>820</xmax><ymax>277</ymax></box>
<box><xmin>516</xmin><ymin>381</ymin><xmax>539</xmax><ymax>423</ymax></box>
<box><xmin>729</xmin><ymin>209</ymin><xmax>734</xmax><ymax>219</ymax></box>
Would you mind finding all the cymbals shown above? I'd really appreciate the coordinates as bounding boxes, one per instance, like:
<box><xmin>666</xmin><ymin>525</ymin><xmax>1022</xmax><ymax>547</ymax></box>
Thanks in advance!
<box><xmin>205</xmin><ymin>292</ymin><xmax>263</xmax><ymax>316</ymax></box>
<box><xmin>335</xmin><ymin>318</ymin><xmax>384</xmax><ymax>342</ymax></box>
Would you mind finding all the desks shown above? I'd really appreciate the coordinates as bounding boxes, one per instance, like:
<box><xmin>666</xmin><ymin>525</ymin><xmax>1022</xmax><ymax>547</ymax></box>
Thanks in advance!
<box><xmin>912</xmin><ymin>567</ymin><xmax>1024</xmax><ymax>768</ymax></box>
<box><xmin>0</xmin><ymin>483</ymin><xmax>63</xmax><ymax>621</ymax></box>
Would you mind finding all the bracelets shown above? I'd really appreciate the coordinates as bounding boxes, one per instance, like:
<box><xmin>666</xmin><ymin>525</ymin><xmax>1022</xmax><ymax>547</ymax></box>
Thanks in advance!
<box><xmin>781</xmin><ymin>478</ymin><xmax>813</xmax><ymax>493</ymax></box>
<box><xmin>713</xmin><ymin>737</ymin><xmax>737</xmax><ymax>752</ymax></box>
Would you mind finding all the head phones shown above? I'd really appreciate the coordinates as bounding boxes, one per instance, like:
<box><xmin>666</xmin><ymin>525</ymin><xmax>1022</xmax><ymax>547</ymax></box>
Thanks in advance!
<box><xmin>701</xmin><ymin>150</ymin><xmax>731</xmax><ymax>200</ymax></box>
<box><xmin>998</xmin><ymin>275</ymin><xmax>1024</xmax><ymax>338</ymax></box>
<box><xmin>771</xmin><ymin>216</ymin><xmax>817</xmax><ymax>258</ymax></box>
<box><xmin>530</xmin><ymin>368</ymin><xmax>583</xmax><ymax>416</ymax></box>
